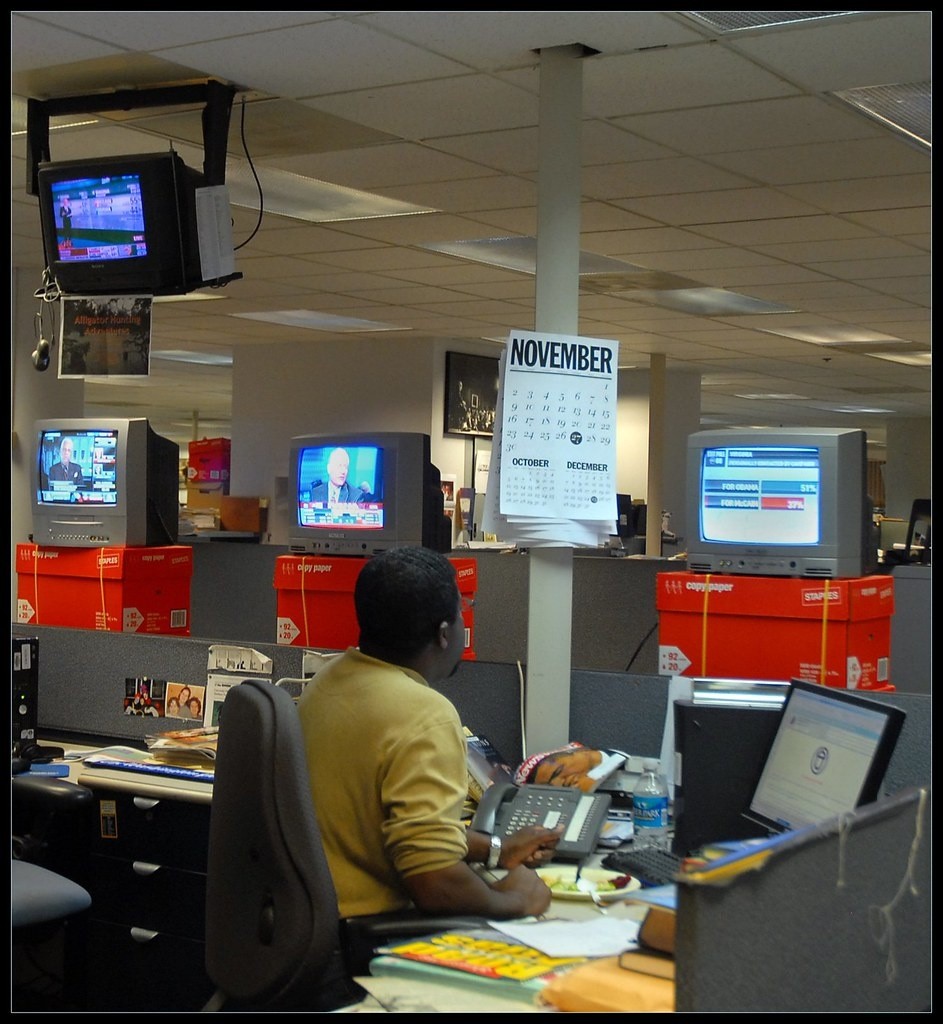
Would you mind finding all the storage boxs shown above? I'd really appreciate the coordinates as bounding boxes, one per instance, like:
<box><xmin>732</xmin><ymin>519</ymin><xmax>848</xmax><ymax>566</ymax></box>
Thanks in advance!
<box><xmin>23</xmin><ymin>543</ymin><xmax>194</xmax><ymax>639</ymax></box>
<box><xmin>221</xmin><ymin>495</ymin><xmax>267</xmax><ymax>533</ymax></box>
<box><xmin>273</xmin><ymin>552</ymin><xmax>480</xmax><ymax>665</ymax></box>
<box><xmin>187</xmin><ymin>482</ymin><xmax>230</xmax><ymax>510</ymax></box>
<box><xmin>652</xmin><ymin>572</ymin><xmax>900</xmax><ymax>695</ymax></box>
<box><xmin>187</xmin><ymin>437</ymin><xmax>229</xmax><ymax>480</ymax></box>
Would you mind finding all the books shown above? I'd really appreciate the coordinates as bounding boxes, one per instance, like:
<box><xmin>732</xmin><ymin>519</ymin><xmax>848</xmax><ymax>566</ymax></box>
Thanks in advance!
<box><xmin>370</xmin><ymin>924</ymin><xmax>587</xmax><ymax>1000</ymax></box>
<box><xmin>460</xmin><ymin>723</ymin><xmax>515</xmax><ymax>804</ymax></box>
<box><xmin>619</xmin><ymin>836</ymin><xmax>761</xmax><ymax>983</ymax></box>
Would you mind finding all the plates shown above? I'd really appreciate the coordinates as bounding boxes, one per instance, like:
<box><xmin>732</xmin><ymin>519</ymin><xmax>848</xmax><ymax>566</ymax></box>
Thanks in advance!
<box><xmin>536</xmin><ymin>868</ymin><xmax>640</xmax><ymax>902</ymax></box>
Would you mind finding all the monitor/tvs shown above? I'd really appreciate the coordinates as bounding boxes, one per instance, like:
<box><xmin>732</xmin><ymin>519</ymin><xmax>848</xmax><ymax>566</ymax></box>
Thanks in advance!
<box><xmin>288</xmin><ymin>431</ymin><xmax>452</xmax><ymax>559</ymax></box>
<box><xmin>686</xmin><ymin>428</ymin><xmax>880</xmax><ymax>580</ymax></box>
<box><xmin>31</xmin><ymin>416</ymin><xmax>179</xmax><ymax>548</ymax></box>
<box><xmin>38</xmin><ymin>151</ymin><xmax>230</xmax><ymax>296</ymax></box>
<box><xmin>740</xmin><ymin>678</ymin><xmax>909</xmax><ymax>838</ymax></box>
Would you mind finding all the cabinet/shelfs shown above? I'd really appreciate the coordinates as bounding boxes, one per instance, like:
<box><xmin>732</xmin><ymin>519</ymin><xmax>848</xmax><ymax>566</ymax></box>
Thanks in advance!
<box><xmin>79</xmin><ymin>769</ymin><xmax>235</xmax><ymax>1011</ymax></box>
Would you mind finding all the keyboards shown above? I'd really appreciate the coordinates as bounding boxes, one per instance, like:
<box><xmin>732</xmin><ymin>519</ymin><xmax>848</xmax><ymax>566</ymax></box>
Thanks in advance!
<box><xmin>601</xmin><ymin>845</ymin><xmax>684</xmax><ymax>887</ymax></box>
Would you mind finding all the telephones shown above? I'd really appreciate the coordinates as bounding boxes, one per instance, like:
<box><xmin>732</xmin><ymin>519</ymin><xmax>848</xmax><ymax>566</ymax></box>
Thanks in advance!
<box><xmin>470</xmin><ymin>781</ymin><xmax>612</xmax><ymax>865</ymax></box>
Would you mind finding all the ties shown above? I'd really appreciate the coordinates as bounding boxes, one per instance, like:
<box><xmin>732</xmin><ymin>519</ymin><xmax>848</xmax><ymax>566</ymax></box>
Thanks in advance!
<box><xmin>62</xmin><ymin>463</ymin><xmax>68</xmax><ymax>480</ymax></box>
<box><xmin>331</xmin><ymin>489</ymin><xmax>336</xmax><ymax>504</ymax></box>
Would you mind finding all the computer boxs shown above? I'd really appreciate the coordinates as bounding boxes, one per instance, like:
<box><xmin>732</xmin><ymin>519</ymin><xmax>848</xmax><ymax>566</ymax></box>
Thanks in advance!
<box><xmin>669</xmin><ymin>699</ymin><xmax>792</xmax><ymax>861</ymax></box>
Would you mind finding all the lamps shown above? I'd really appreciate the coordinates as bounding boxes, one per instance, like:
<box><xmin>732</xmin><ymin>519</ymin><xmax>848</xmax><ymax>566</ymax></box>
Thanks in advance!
<box><xmin>32</xmin><ymin>290</ymin><xmax>55</xmax><ymax>371</ymax></box>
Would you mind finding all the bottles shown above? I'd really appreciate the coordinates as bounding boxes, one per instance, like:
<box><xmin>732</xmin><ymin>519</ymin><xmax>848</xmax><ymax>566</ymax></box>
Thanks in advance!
<box><xmin>632</xmin><ymin>758</ymin><xmax>668</xmax><ymax>852</ymax></box>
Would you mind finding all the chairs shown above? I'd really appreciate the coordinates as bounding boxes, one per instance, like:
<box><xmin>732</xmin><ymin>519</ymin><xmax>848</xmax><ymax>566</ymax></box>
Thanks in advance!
<box><xmin>11</xmin><ymin>772</ymin><xmax>93</xmax><ymax>930</ymax></box>
<box><xmin>206</xmin><ymin>681</ymin><xmax>491</xmax><ymax>1012</ymax></box>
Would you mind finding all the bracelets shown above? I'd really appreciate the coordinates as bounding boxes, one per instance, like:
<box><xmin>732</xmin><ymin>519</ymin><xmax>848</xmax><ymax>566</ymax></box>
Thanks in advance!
<box><xmin>486</xmin><ymin>834</ymin><xmax>502</xmax><ymax>869</ymax></box>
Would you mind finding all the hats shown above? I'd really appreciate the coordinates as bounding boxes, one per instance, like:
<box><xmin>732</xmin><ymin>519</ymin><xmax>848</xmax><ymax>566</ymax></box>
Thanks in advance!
<box><xmin>142</xmin><ymin>685</ymin><xmax>148</xmax><ymax>692</ymax></box>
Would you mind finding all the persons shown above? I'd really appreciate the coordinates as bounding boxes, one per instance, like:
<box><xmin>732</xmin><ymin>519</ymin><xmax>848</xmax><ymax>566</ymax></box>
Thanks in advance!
<box><xmin>188</xmin><ymin>696</ymin><xmax>202</xmax><ymax>720</ymax></box>
<box><xmin>452</xmin><ymin>380</ymin><xmax>495</xmax><ymax>432</ymax></box>
<box><xmin>64</xmin><ymin>298</ymin><xmax>151</xmax><ymax>373</ymax></box>
<box><xmin>60</xmin><ymin>198</ymin><xmax>72</xmax><ymax>244</ymax></box>
<box><xmin>167</xmin><ymin>697</ymin><xmax>180</xmax><ymax>717</ymax></box>
<box><xmin>125</xmin><ymin>685</ymin><xmax>164</xmax><ymax>717</ymax></box>
<box><xmin>298</xmin><ymin>544</ymin><xmax>561</xmax><ymax>991</ymax></box>
<box><xmin>178</xmin><ymin>687</ymin><xmax>191</xmax><ymax>718</ymax></box>
<box><xmin>49</xmin><ymin>437</ymin><xmax>83</xmax><ymax>485</ymax></box>
<box><xmin>310</xmin><ymin>448</ymin><xmax>380</xmax><ymax>502</ymax></box>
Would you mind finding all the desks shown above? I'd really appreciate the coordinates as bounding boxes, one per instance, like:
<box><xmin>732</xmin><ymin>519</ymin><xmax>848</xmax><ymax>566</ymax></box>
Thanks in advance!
<box><xmin>341</xmin><ymin>730</ymin><xmax>741</xmax><ymax>1011</ymax></box>
